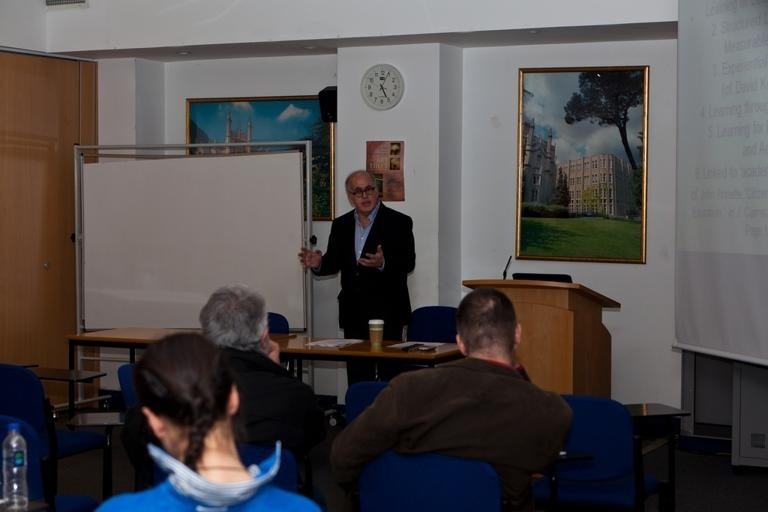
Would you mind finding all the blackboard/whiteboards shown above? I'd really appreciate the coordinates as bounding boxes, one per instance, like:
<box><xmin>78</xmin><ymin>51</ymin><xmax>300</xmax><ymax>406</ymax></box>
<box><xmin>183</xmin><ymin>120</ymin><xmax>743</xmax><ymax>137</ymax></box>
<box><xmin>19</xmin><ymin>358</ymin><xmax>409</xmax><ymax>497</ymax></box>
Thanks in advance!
<box><xmin>79</xmin><ymin>150</ymin><xmax>306</xmax><ymax>333</ymax></box>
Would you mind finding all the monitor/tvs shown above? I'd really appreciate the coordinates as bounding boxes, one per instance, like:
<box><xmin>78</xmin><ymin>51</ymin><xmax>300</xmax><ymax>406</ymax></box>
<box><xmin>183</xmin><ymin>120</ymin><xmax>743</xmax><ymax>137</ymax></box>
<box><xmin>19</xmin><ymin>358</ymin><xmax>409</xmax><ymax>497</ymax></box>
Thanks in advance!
<box><xmin>512</xmin><ymin>272</ymin><xmax>573</xmax><ymax>284</ymax></box>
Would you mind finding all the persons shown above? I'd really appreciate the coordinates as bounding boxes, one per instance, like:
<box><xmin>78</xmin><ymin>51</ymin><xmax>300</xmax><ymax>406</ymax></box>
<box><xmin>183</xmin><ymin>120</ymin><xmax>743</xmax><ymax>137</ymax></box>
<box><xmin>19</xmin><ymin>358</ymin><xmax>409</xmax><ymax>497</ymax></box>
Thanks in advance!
<box><xmin>294</xmin><ymin>168</ymin><xmax>415</xmax><ymax>386</ymax></box>
<box><xmin>326</xmin><ymin>286</ymin><xmax>577</xmax><ymax>511</ymax></box>
<box><xmin>94</xmin><ymin>330</ymin><xmax>326</xmax><ymax>512</ymax></box>
<box><xmin>117</xmin><ymin>283</ymin><xmax>327</xmax><ymax>485</ymax></box>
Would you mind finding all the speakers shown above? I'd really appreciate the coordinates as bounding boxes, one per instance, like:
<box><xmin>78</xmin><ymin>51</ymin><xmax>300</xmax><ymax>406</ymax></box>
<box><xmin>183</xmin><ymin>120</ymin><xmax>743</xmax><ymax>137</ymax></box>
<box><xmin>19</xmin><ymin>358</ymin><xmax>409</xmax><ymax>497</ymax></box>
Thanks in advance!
<box><xmin>319</xmin><ymin>86</ymin><xmax>336</xmax><ymax>122</ymax></box>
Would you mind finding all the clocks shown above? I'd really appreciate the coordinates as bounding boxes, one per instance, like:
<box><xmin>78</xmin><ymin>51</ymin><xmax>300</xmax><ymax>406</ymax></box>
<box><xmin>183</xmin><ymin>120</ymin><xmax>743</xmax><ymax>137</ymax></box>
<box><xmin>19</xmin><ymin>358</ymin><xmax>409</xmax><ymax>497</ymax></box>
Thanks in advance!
<box><xmin>358</xmin><ymin>62</ymin><xmax>405</xmax><ymax>110</ymax></box>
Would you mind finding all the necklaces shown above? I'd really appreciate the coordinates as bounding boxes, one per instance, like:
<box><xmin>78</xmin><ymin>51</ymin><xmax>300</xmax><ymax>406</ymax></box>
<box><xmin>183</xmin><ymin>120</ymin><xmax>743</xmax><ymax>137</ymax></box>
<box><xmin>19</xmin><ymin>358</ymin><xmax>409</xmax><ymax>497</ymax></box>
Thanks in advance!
<box><xmin>193</xmin><ymin>466</ymin><xmax>248</xmax><ymax>471</ymax></box>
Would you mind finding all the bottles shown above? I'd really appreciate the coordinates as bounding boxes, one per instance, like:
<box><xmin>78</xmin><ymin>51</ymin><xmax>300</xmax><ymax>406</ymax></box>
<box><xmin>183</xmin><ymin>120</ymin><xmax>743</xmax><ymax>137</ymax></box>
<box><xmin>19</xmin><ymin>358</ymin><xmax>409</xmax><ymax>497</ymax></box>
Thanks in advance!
<box><xmin>2</xmin><ymin>422</ymin><xmax>29</xmax><ymax>511</ymax></box>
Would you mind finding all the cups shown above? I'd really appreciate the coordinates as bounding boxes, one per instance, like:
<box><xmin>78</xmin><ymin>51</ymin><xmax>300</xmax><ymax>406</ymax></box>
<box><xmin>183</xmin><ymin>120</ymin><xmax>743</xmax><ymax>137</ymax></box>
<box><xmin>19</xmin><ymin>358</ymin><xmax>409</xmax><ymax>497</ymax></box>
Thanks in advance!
<box><xmin>368</xmin><ymin>320</ymin><xmax>384</xmax><ymax>346</ymax></box>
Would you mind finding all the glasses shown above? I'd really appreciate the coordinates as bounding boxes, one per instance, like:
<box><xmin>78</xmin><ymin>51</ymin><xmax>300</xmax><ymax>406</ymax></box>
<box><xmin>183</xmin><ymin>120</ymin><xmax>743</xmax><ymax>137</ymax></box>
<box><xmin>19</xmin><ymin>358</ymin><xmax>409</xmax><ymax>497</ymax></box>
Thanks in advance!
<box><xmin>347</xmin><ymin>186</ymin><xmax>377</xmax><ymax>198</ymax></box>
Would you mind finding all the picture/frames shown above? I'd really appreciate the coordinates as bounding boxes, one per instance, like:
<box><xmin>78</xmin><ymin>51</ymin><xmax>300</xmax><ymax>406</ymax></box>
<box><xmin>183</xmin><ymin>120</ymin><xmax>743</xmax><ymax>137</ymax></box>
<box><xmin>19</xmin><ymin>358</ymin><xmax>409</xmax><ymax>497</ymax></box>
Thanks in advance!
<box><xmin>182</xmin><ymin>93</ymin><xmax>333</xmax><ymax>222</ymax></box>
<box><xmin>513</xmin><ymin>63</ymin><xmax>648</xmax><ymax>266</ymax></box>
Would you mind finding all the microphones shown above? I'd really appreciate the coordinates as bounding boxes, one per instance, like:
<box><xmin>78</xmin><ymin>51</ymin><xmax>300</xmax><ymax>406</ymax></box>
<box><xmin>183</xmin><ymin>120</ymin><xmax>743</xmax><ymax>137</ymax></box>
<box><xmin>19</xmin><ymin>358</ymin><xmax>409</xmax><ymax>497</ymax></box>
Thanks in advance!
<box><xmin>501</xmin><ymin>255</ymin><xmax>512</xmax><ymax>279</ymax></box>
